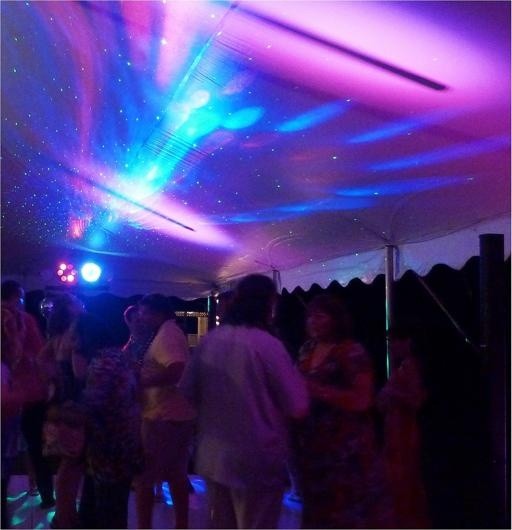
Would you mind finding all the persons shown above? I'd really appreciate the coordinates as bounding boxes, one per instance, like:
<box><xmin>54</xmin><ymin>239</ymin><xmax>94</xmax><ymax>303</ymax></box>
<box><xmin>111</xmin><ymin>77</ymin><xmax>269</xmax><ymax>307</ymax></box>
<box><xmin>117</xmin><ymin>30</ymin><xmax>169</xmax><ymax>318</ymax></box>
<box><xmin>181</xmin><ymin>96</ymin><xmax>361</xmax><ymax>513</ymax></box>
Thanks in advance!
<box><xmin>1</xmin><ymin>273</ymin><xmax>431</xmax><ymax>529</ymax></box>
